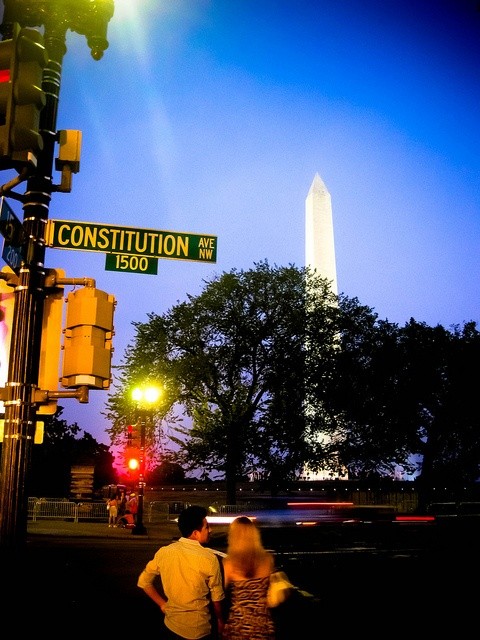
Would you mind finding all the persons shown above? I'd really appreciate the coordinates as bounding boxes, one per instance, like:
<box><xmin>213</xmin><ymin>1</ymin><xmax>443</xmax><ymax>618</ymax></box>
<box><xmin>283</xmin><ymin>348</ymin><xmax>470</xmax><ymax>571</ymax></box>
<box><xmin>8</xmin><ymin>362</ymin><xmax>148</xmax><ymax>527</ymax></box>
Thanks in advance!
<box><xmin>106</xmin><ymin>489</ymin><xmax>141</xmax><ymax>529</ymax></box>
<box><xmin>136</xmin><ymin>506</ymin><xmax>224</xmax><ymax>639</ymax></box>
<box><xmin>222</xmin><ymin>516</ymin><xmax>290</xmax><ymax>639</ymax></box>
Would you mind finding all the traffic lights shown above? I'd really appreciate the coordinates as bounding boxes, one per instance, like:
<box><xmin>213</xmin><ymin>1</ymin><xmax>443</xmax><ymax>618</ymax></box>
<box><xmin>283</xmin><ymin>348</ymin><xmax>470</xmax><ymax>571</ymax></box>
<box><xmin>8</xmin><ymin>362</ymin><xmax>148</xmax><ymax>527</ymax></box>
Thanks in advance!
<box><xmin>0</xmin><ymin>21</ymin><xmax>49</xmax><ymax>163</ymax></box>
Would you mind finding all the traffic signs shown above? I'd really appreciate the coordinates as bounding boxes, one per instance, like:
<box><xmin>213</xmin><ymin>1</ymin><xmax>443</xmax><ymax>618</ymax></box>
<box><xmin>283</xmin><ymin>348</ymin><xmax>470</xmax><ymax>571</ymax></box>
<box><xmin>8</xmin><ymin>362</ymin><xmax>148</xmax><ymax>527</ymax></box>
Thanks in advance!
<box><xmin>52</xmin><ymin>218</ymin><xmax>218</xmax><ymax>276</ymax></box>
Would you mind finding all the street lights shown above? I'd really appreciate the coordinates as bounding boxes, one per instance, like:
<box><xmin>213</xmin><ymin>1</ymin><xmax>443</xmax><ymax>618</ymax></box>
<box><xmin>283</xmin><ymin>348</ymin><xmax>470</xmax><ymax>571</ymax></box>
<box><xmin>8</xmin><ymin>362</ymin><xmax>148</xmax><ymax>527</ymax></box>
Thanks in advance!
<box><xmin>131</xmin><ymin>386</ymin><xmax>162</xmax><ymax>535</ymax></box>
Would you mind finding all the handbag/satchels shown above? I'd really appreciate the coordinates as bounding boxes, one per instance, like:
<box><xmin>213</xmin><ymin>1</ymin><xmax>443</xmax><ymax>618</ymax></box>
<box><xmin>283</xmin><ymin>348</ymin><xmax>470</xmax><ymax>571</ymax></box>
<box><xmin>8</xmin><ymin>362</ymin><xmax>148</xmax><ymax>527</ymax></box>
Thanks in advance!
<box><xmin>265</xmin><ymin>571</ymin><xmax>292</xmax><ymax>609</ymax></box>
